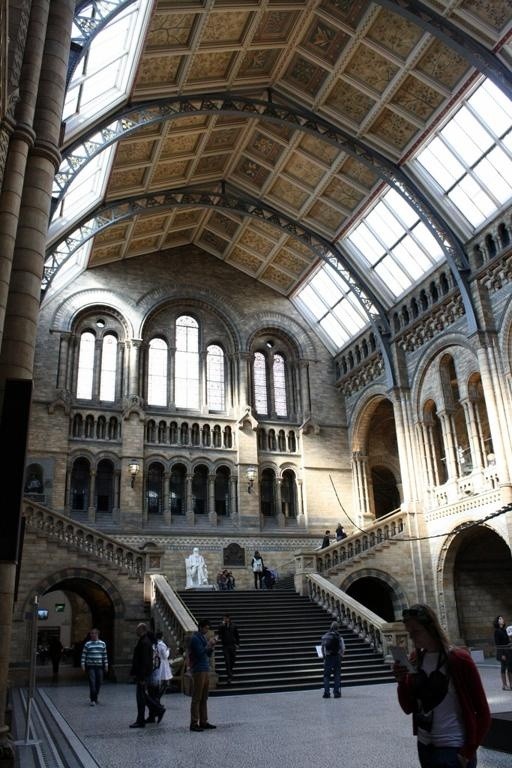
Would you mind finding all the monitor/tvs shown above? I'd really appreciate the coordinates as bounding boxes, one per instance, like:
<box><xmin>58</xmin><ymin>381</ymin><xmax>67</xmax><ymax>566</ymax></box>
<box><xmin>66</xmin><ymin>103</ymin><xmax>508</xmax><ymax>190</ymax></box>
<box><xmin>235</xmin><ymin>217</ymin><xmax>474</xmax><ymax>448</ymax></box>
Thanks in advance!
<box><xmin>38</xmin><ymin>608</ymin><xmax>49</xmax><ymax>619</ymax></box>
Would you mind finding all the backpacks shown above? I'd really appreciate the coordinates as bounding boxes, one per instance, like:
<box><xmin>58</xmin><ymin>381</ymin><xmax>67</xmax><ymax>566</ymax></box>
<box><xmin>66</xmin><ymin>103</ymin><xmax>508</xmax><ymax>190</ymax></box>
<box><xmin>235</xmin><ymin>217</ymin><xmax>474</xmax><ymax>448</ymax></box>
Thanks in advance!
<box><xmin>253</xmin><ymin>557</ymin><xmax>262</xmax><ymax>573</ymax></box>
<box><xmin>323</xmin><ymin>633</ymin><xmax>340</xmax><ymax>653</ymax></box>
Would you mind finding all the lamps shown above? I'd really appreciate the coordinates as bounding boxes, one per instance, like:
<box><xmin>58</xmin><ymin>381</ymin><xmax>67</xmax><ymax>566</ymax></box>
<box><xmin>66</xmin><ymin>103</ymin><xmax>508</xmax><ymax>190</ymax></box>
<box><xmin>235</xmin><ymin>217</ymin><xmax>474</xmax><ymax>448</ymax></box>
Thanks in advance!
<box><xmin>245</xmin><ymin>465</ymin><xmax>257</xmax><ymax>494</ymax></box>
<box><xmin>127</xmin><ymin>457</ymin><xmax>141</xmax><ymax>487</ymax></box>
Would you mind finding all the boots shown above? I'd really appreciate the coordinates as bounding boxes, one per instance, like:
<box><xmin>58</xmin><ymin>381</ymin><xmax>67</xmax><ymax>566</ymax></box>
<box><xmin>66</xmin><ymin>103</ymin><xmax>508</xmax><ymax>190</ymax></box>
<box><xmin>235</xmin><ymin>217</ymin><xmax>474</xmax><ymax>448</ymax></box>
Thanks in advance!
<box><xmin>501</xmin><ymin>672</ymin><xmax>512</xmax><ymax>690</ymax></box>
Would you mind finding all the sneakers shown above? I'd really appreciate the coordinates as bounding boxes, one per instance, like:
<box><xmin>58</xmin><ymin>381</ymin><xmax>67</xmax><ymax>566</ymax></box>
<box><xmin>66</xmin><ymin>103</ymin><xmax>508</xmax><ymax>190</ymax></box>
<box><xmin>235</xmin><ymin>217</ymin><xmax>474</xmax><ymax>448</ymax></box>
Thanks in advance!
<box><xmin>200</xmin><ymin>722</ymin><xmax>216</xmax><ymax>729</ymax></box>
<box><xmin>129</xmin><ymin>707</ymin><xmax>166</xmax><ymax>728</ymax></box>
<box><xmin>190</xmin><ymin>724</ymin><xmax>204</xmax><ymax>732</ymax></box>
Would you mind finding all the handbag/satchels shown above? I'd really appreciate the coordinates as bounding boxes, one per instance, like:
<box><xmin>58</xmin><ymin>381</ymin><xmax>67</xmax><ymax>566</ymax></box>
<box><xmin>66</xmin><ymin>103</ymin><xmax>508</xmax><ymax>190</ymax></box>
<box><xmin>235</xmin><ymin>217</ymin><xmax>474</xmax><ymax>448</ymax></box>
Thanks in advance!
<box><xmin>152</xmin><ymin>656</ymin><xmax>160</xmax><ymax>671</ymax></box>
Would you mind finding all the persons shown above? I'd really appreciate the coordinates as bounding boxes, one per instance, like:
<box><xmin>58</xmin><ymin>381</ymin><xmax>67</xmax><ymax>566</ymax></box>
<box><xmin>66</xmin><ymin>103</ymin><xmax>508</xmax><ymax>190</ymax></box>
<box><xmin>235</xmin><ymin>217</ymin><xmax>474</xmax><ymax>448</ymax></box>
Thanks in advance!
<box><xmin>80</xmin><ymin>628</ymin><xmax>109</xmax><ymax>706</ymax></box>
<box><xmin>217</xmin><ymin>569</ymin><xmax>235</xmax><ymax>591</ymax></box>
<box><xmin>320</xmin><ymin>620</ymin><xmax>345</xmax><ymax>698</ymax></box>
<box><xmin>127</xmin><ymin>614</ymin><xmax>216</xmax><ymax>732</ymax></box>
<box><xmin>49</xmin><ymin>634</ymin><xmax>63</xmax><ymax>678</ymax></box>
<box><xmin>188</xmin><ymin>547</ymin><xmax>208</xmax><ymax>585</ymax></box>
<box><xmin>252</xmin><ymin>550</ymin><xmax>264</xmax><ymax>589</ymax></box>
<box><xmin>493</xmin><ymin>615</ymin><xmax>512</xmax><ymax>690</ymax></box>
<box><xmin>218</xmin><ymin>615</ymin><xmax>241</xmax><ymax>683</ymax></box>
<box><xmin>336</xmin><ymin>523</ymin><xmax>345</xmax><ymax>542</ymax></box>
<box><xmin>322</xmin><ymin>530</ymin><xmax>330</xmax><ymax>549</ymax></box>
<box><xmin>392</xmin><ymin>603</ymin><xmax>492</xmax><ymax>768</ymax></box>
